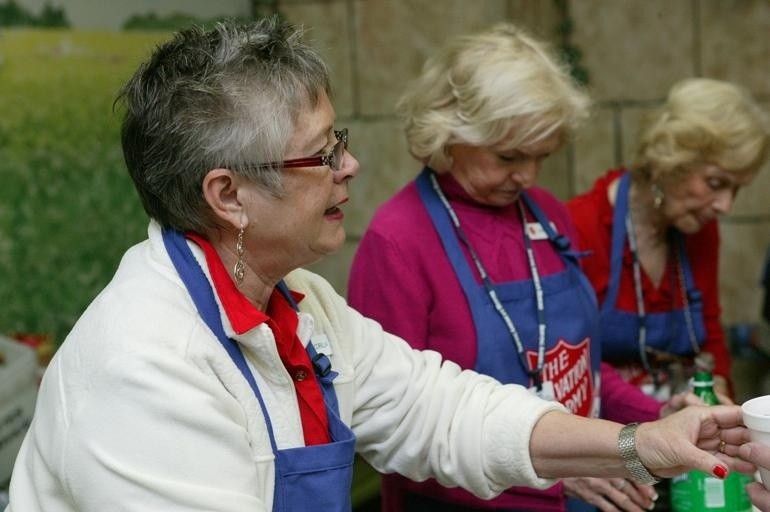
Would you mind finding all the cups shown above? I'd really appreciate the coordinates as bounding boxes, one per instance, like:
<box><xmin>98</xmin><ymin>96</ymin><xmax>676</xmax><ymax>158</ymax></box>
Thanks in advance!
<box><xmin>740</xmin><ymin>395</ymin><xmax>770</xmax><ymax>492</ymax></box>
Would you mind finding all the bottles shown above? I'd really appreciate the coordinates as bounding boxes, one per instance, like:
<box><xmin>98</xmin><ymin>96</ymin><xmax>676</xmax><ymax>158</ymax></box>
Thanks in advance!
<box><xmin>671</xmin><ymin>371</ymin><xmax>753</xmax><ymax>512</ymax></box>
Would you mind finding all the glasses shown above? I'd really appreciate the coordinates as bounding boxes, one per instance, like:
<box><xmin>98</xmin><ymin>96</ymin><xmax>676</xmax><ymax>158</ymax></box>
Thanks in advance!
<box><xmin>224</xmin><ymin>129</ymin><xmax>349</xmax><ymax>170</ymax></box>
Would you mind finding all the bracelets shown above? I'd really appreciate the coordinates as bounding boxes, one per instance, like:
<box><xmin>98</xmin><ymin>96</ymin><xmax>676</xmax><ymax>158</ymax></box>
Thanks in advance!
<box><xmin>618</xmin><ymin>422</ymin><xmax>663</xmax><ymax>487</ymax></box>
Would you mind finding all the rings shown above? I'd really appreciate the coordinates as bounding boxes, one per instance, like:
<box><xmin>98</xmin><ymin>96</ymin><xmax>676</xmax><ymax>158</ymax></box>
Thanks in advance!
<box><xmin>719</xmin><ymin>440</ymin><xmax>726</xmax><ymax>453</ymax></box>
<box><xmin>618</xmin><ymin>478</ymin><xmax>627</xmax><ymax>491</ymax></box>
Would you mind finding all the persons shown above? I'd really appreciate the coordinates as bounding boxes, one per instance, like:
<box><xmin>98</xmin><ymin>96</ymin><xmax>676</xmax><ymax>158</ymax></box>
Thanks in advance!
<box><xmin>561</xmin><ymin>78</ymin><xmax>770</xmax><ymax>397</ymax></box>
<box><xmin>349</xmin><ymin>23</ymin><xmax>733</xmax><ymax>511</ymax></box>
<box><xmin>0</xmin><ymin>12</ymin><xmax>755</xmax><ymax>512</ymax></box>
<box><xmin>739</xmin><ymin>440</ymin><xmax>770</xmax><ymax>512</ymax></box>
